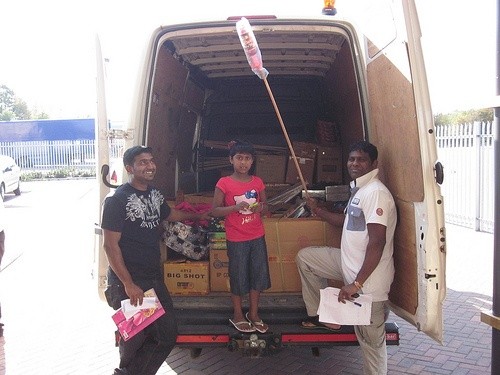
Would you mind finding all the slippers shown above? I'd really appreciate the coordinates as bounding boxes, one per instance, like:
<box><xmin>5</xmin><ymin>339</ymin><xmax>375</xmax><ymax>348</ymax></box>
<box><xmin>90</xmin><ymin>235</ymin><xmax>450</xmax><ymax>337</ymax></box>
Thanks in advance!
<box><xmin>297</xmin><ymin>316</ymin><xmax>342</xmax><ymax>332</ymax></box>
<box><xmin>229</xmin><ymin>318</ymin><xmax>257</xmax><ymax>332</ymax></box>
<box><xmin>245</xmin><ymin>311</ymin><xmax>269</xmax><ymax>334</ymax></box>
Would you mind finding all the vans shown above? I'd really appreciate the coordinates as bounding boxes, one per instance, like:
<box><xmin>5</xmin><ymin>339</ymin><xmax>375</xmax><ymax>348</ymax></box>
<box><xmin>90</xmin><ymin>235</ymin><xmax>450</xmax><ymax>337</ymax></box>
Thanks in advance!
<box><xmin>95</xmin><ymin>1</ymin><xmax>447</xmax><ymax>359</ymax></box>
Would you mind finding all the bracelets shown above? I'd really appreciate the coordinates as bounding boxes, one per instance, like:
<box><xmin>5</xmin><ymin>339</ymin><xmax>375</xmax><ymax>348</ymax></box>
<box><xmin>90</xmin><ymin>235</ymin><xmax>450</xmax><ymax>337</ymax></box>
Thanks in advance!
<box><xmin>353</xmin><ymin>281</ymin><xmax>363</xmax><ymax>290</ymax></box>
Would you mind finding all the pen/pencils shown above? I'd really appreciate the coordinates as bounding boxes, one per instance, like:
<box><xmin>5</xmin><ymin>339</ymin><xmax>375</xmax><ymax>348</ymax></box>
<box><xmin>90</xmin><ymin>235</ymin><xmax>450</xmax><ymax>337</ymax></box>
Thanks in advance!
<box><xmin>333</xmin><ymin>293</ymin><xmax>362</xmax><ymax>307</ymax></box>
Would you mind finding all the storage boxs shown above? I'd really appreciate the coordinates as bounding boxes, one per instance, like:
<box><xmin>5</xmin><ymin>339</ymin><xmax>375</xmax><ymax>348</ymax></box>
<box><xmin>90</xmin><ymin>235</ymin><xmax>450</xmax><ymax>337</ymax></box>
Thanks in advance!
<box><xmin>162</xmin><ymin>141</ymin><xmax>347</xmax><ymax>295</ymax></box>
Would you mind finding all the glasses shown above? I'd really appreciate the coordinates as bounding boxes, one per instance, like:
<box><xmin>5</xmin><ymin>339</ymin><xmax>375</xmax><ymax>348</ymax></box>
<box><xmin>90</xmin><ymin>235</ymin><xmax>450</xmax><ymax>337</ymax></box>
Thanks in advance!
<box><xmin>130</xmin><ymin>158</ymin><xmax>159</xmax><ymax>165</ymax></box>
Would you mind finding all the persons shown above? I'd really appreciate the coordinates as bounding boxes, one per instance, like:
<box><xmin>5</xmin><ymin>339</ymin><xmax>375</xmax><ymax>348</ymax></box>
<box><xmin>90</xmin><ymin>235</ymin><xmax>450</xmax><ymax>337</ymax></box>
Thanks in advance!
<box><xmin>101</xmin><ymin>146</ymin><xmax>214</xmax><ymax>375</ymax></box>
<box><xmin>296</xmin><ymin>140</ymin><xmax>397</xmax><ymax>375</ymax></box>
<box><xmin>212</xmin><ymin>143</ymin><xmax>272</xmax><ymax>333</ymax></box>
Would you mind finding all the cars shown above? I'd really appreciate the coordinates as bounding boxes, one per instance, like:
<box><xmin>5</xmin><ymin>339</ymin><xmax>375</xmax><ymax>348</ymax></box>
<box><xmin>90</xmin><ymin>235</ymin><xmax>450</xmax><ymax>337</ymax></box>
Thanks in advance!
<box><xmin>0</xmin><ymin>154</ymin><xmax>22</xmax><ymax>203</ymax></box>
